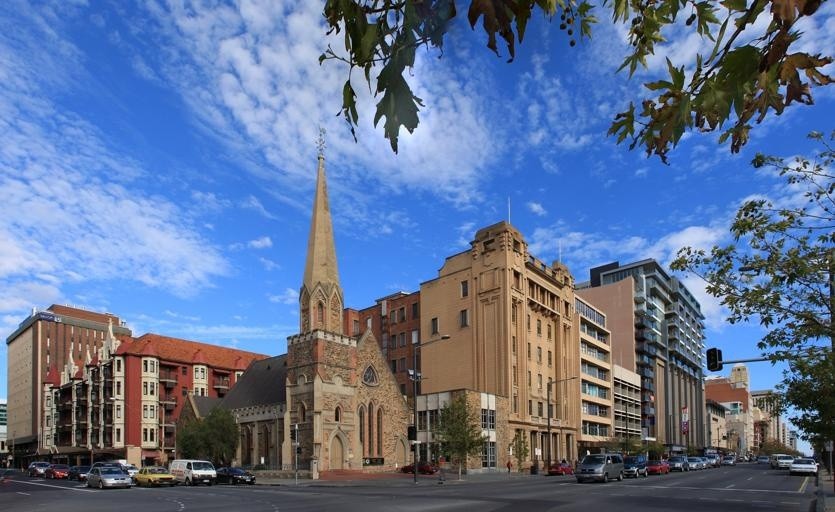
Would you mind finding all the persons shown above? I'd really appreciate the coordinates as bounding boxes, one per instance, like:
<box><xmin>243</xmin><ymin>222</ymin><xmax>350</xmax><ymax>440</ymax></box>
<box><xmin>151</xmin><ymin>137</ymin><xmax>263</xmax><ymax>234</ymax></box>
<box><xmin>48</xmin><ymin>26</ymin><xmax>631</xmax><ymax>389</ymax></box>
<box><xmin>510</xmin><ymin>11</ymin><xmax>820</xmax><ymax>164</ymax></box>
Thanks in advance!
<box><xmin>507</xmin><ymin>460</ymin><xmax>512</xmax><ymax>473</ymax></box>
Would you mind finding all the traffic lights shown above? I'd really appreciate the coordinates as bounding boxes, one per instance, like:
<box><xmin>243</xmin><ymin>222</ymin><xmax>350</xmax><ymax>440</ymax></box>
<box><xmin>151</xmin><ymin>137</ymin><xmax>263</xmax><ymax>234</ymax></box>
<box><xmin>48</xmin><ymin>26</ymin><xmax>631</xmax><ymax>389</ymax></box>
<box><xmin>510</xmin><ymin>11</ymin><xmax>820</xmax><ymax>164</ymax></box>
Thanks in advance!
<box><xmin>410</xmin><ymin>444</ymin><xmax>415</xmax><ymax>451</ymax></box>
<box><xmin>707</xmin><ymin>348</ymin><xmax>719</xmax><ymax>370</ymax></box>
<box><xmin>296</xmin><ymin>447</ymin><xmax>301</xmax><ymax>455</ymax></box>
<box><xmin>408</xmin><ymin>426</ymin><xmax>416</xmax><ymax>440</ymax></box>
<box><xmin>289</xmin><ymin>428</ymin><xmax>296</xmax><ymax>440</ymax></box>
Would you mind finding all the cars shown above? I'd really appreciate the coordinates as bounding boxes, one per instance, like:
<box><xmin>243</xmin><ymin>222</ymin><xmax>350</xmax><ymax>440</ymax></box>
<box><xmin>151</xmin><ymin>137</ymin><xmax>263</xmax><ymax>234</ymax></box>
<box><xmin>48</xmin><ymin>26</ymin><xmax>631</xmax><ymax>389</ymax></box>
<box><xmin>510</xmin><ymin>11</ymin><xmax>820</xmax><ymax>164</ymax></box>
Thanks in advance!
<box><xmin>400</xmin><ymin>460</ymin><xmax>440</xmax><ymax>474</ymax></box>
<box><xmin>771</xmin><ymin>454</ymin><xmax>795</xmax><ymax>469</ymax></box>
<box><xmin>545</xmin><ymin>462</ymin><xmax>574</xmax><ymax>476</ymax></box>
<box><xmin>788</xmin><ymin>457</ymin><xmax>820</xmax><ymax>476</ymax></box>
<box><xmin>26</xmin><ymin>458</ymin><xmax>256</xmax><ymax>488</ymax></box>
<box><xmin>646</xmin><ymin>453</ymin><xmax>772</xmax><ymax>475</ymax></box>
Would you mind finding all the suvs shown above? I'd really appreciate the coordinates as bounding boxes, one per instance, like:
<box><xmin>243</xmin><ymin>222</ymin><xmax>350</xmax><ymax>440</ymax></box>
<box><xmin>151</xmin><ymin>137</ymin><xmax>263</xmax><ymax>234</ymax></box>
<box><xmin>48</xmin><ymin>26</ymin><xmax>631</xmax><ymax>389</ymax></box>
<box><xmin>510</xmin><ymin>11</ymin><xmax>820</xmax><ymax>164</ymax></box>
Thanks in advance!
<box><xmin>623</xmin><ymin>455</ymin><xmax>649</xmax><ymax>478</ymax></box>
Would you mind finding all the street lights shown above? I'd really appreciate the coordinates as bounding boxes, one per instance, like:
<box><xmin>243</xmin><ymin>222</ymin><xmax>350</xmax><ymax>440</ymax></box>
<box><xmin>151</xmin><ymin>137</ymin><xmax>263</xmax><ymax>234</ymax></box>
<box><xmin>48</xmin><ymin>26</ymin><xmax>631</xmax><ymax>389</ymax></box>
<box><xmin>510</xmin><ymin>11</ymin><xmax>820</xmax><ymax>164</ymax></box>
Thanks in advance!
<box><xmin>109</xmin><ymin>397</ymin><xmax>165</xmax><ymax>466</ymax></box>
<box><xmin>407</xmin><ymin>334</ymin><xmax>450</xmax><ymax>484</ymax></box>
<box><xmin>547</xmin><ymin>375</ymin><xmax>578</xmax><ymax>466</ymax></box>
<box><xmin>626</xmin><ymin>400</ymin><xmax>649</xmax><ymax>456</ymax></box>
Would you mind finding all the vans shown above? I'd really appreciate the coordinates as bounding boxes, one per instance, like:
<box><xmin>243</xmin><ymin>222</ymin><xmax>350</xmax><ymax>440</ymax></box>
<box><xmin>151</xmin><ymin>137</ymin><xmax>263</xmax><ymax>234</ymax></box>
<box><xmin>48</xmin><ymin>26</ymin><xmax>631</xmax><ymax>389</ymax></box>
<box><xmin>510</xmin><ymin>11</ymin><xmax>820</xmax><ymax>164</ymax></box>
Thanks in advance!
<box><xmin>574</xmin><ymin>453</ymin><xmax>624</xmax><ymax>483</ymax></box>
